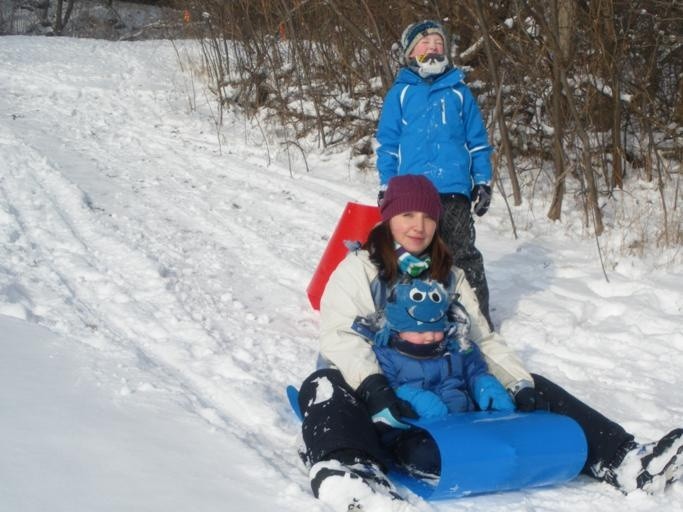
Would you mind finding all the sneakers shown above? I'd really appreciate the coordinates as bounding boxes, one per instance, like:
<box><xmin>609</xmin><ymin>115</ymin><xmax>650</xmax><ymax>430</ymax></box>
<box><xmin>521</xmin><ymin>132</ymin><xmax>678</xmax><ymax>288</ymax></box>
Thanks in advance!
<box><xmin>314</xmin><ymin>463</ymin><xmax>399</xmax><ymax>509</ymax></box>
<box><xmin>611</xmin><ymin>430</ymin><xmax>683</xmax><ymax>493</ymax></box>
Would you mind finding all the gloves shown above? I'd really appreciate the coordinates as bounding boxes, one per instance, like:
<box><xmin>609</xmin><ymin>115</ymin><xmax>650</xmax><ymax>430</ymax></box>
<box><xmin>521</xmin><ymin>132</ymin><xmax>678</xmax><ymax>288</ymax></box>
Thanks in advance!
<box><xmin>468</xmin><ymin>375</ymin><xmax>517</xmax><ymax>412</ymax></box>
<box><xmin>471</xmin><ymin>185</ymin><xmax>491</xmax><ymax>216</ymax></box>
<box><xmin>355</xmin><ymin>375</ymin><xmax>418</xmax><ymax>430</ymax></box>
<box><xmin>376</xmin><ymin>189</ymin><xmax>386</xmax><ymax>204</ymax></box>
<box><xmin>396</xmin><ymin>384</ymin><xmax>448</xmax><ymax>417</ymax></box>
<box><xmin>513</xmin><ymin>387</ymin><xmax>554</xmax><ymax>411</ymax></box>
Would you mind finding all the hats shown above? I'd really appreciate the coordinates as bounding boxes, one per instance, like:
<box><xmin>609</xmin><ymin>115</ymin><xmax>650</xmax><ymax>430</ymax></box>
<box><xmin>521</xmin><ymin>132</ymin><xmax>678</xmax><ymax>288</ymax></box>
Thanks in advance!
<box><xmin>385</xmin><ymin>277</ymin><xmax>455</xmax><ymax>336</ymax></box>
<box><xmin>381</xmin><ymin>175</ymin><xmax>442</xmax><ymax>224</ymax></box>
<box><xmin>402</xmin><ymin>22</ymin><xmax>444</xmax><ymax>57</ymax></box>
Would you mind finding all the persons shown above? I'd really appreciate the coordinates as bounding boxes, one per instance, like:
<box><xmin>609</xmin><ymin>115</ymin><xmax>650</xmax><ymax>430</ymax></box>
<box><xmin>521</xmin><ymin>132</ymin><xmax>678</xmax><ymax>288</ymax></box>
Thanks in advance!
<box><xmin>371</xmin><ymin>19</ymin><xmax>497</xmax><ymax>337</ymax></box>
<box><xmin>372</xmin><ymin>278</ymin><xmax>518</xmax><ymax>418</ymax></box>
<box><xmin>297</xmin><ymin>173</ymin><xmax>682</xmax><ymax>512</ymax></box>
